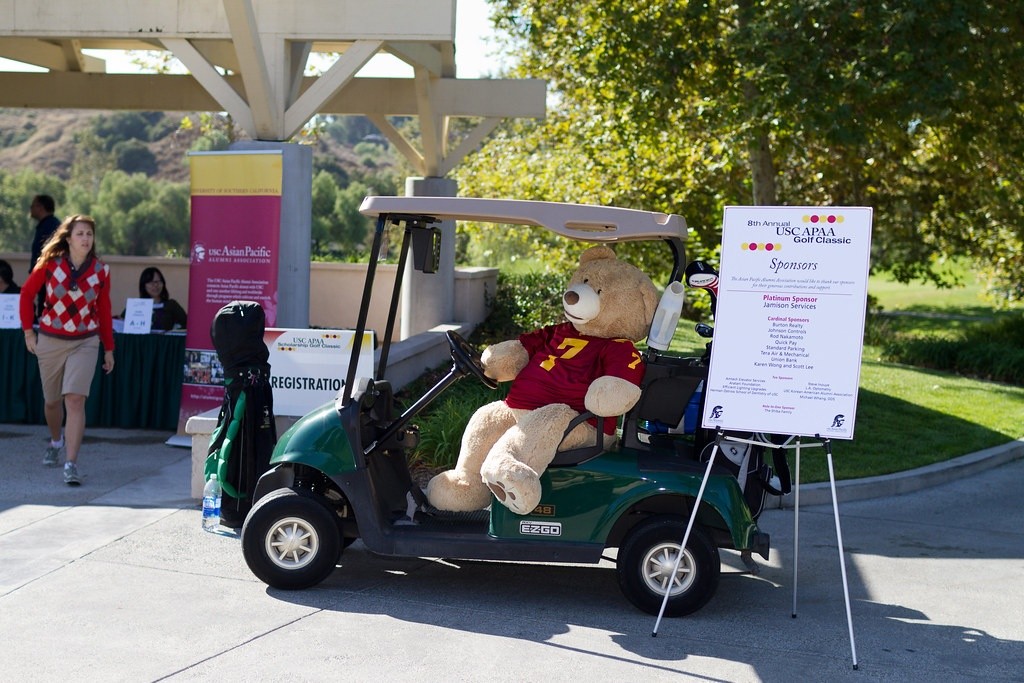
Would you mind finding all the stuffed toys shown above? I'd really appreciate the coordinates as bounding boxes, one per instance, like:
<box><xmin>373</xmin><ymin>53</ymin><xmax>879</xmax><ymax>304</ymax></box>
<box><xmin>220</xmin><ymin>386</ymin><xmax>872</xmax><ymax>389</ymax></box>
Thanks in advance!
<box><xmin>428</xmin><ymin>245</ymin><xmax>660</xmax><ymax>514</ymax></box>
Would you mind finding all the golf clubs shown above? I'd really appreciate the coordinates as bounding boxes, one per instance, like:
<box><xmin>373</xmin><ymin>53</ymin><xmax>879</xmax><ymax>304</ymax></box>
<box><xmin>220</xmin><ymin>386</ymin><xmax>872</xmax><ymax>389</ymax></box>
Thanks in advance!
<box><xmin>694</xmin><ymin>323</ymin><xmax>714</xmax><ymax>338</ymax></box>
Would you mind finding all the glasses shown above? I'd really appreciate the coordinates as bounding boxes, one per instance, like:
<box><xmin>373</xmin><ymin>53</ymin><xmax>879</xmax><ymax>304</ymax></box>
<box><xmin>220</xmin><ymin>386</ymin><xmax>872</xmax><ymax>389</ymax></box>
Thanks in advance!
<box><xmin>146</xmin><ymin>280</ymin><xmax>162</xmax><ymax>283</ymax></box>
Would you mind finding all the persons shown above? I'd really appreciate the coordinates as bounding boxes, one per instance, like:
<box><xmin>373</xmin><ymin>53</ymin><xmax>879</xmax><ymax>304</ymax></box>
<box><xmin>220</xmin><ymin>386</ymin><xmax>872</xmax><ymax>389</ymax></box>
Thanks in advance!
<box><xmin>27</xmin><ymin>193</ymin><xmax>62</xmax><ymax>323</ymax></box>
<box><xmin>120</xmin><ymin>266</ymin><xmax>187</xmax><ymax>330</ymax></box>
<box><xmin>18</xmin><ymin>213</ymin><xmax>115</xmax><ymax>485</ymax></box>
<box><xmin>0</xmin><ymin>258</ymin><xmax>20</xmax><ymax>294</ymax></box>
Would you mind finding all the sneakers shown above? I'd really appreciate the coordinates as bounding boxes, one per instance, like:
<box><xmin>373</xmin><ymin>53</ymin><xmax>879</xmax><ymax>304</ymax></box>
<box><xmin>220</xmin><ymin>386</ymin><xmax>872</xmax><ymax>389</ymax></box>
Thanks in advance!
<box><xmin>43</xmin><ymin>435</ymin><xmax>66</xmax><ymax>465</ymax></box>
<box><xmin>63</xmin><ymin>461</ymin><xmax>80</xmax><ymax>485</ymax></box>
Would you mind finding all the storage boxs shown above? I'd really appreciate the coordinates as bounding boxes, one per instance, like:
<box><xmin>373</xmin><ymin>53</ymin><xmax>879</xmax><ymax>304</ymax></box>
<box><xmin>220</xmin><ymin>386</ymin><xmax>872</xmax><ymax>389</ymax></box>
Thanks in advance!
<box><xmin>637</xmin><ymin>355</ymin><xmax>705</xmax><ymax>428</ymax></box>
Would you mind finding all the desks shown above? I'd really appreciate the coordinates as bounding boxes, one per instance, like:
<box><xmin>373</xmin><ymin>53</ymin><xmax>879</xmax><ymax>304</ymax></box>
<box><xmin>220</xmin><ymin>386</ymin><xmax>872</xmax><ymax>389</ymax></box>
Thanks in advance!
<box><xmin>1</xmin><ymin>325</ymin><xmax>187</xmax><ymax>432</ymax></box>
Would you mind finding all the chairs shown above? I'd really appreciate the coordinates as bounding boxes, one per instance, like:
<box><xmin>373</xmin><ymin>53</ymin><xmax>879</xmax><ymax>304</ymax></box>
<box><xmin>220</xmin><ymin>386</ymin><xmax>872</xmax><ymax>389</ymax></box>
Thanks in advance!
<box><xmin>547</xmin><ymin>349</ymin><xmax>647</xmax><ymax>468</ymax></box>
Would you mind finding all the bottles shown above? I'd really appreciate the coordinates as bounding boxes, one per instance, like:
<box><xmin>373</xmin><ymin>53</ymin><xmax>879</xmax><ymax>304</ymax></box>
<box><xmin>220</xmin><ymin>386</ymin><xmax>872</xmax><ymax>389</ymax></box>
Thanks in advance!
<box><xmin>201</xmin><ymin>473</ymin><xmax>223</xmax><ymax>532</ymax></box>
